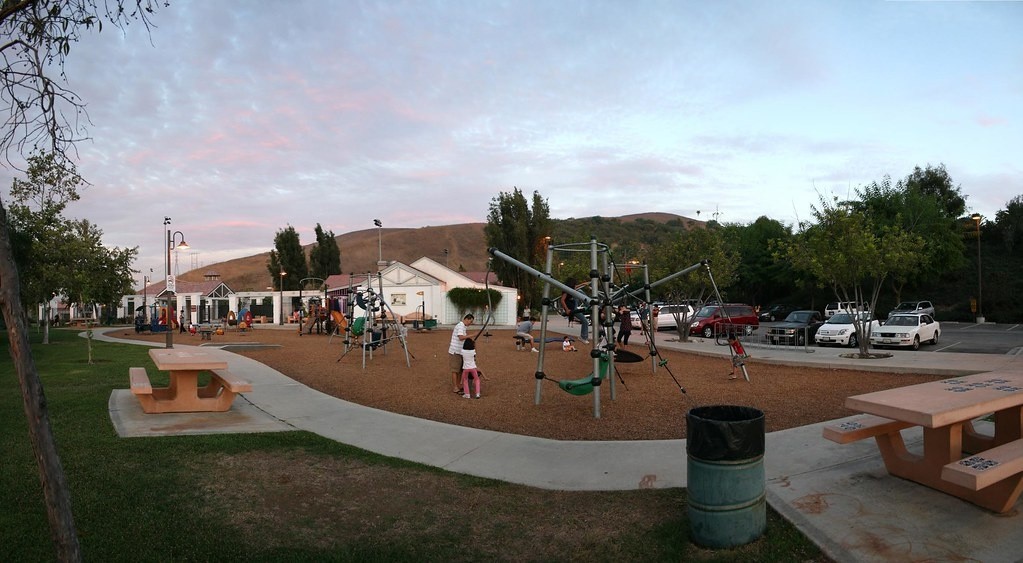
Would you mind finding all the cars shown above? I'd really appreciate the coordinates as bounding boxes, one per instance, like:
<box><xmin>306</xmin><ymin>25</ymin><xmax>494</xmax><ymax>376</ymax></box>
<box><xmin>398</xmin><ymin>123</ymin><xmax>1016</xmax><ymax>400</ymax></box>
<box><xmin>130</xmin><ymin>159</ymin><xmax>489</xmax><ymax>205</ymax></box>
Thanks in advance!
<box><xmin>758</xmin><ymin>304</ymin><xmax>803</xmax><ymax>322</ymax></box>
<box><xmin>815</xmin><ymin>311</ymin><xmax>880</xmax><ymax>348</ymax></box>
<box><xmin>870</xmin><ymin>313</ymin><xmax>941</xmax><ymax>351</ymax></box>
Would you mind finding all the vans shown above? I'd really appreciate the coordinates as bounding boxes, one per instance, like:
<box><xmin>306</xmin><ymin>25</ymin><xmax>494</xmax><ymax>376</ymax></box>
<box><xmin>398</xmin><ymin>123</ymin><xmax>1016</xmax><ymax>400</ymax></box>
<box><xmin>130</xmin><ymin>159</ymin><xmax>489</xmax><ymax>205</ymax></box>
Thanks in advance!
<box><xmin>824</xmin><ymin>301</ymin><xmax>871</xmax><ymax>319</ymax></box>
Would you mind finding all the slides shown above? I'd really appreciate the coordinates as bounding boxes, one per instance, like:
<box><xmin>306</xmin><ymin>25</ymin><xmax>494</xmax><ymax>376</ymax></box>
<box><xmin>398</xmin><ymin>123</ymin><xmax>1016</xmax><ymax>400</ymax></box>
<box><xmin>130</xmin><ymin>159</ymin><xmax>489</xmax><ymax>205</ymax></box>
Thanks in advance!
<box><xmin>302</xmin><ymin>316</ymin><xmax>317</xmax><ymax>334</ymax></box>
<box><xmin>331</xmin><ymin>309</ymin><xmax>348</xmax><ymax>334</ymax></box>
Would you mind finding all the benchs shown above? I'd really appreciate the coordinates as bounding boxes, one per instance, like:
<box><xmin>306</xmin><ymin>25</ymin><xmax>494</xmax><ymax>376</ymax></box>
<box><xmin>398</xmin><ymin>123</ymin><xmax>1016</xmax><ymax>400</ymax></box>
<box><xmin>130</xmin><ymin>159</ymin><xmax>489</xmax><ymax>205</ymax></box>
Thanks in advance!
<box><xmin>81</xmin><ymin>322</ymin><xmax>98</xmax><ymax>328</ymax></box>
<box><xmin>209</xmin><ymin>370</ymin><xmax>253</xmax><ymax>412</ymax></box>
<box><xmin>822</xmin><ymin>415</ymin><xmax>918</xmax><ymax>445</ymax></box>
<box><xmin>941</xmin><ymin>438</ymin><xmax>1023</xmax><ymax>491</ymax></box>
<box><xmin>64</xmin><ymin>322</ymin><xmax>77</xmax><ymax>327</ymax></box>
<box><xmin>129</xmin><ymin>367</ymin><xmax>152</xmax><ymax>414</ymax></box>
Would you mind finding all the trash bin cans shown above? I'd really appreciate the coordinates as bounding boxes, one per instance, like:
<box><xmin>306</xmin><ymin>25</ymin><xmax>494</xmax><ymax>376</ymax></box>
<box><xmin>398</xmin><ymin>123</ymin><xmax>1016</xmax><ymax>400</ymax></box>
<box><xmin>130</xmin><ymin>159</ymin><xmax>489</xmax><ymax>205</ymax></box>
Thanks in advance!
<box><xmin>684</xmin><ymin>403</ymin><xmax>767</xmax><ymax>549</ymax></box>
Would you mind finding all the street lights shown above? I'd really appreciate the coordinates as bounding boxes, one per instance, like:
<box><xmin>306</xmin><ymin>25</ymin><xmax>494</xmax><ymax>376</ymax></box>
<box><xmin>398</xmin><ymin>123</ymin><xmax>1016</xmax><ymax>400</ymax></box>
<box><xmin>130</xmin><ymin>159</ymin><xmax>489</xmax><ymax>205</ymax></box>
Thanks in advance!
<box><xmin>972</xmin><ymin>214</ymin><xmax>983</xmax><ymax>323</ymax></box>
<box><xmin>280</xmin><ymin>265</ymin><xmax>288</xmax><ymax>324</ymax></box>
<box><xmin>374</xmin><ymin>219</ymin><xmax>383</xmax><ymax>261</ymax></box>
<box><xmin>167</xmin><ymin>230</ymin><xmax>188</xmax><ymax>348</ymax></box>
<box><xmin>163</xmin><ymin>216</ymin><xmax>171</xmax><ymax>280</ymax></box>
<box><xmin>143</xmin><ymin>276</ymin><xmax>150</xmax><ymax>324</ymax></box>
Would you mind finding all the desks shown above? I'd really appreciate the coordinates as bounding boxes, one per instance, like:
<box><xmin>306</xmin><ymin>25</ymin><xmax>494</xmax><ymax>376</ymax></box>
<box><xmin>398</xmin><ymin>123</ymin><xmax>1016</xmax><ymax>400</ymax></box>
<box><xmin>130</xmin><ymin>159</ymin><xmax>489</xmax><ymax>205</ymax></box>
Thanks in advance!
<box><xmin>148</xmin><ymin>346</ymin><xmax>228</xmax><ymax>412</ymax></box>
<box><xmin>252</xmin><ymin>318</ymin><xmax>261</xmax><ymax>322</ymax></box>
<box><xmin>846</xmin><ymin>368</ymin><xmax>1023</xmax><ymax>513</ymax></box>
<box><xmin>70</xmin><ymin>317</ymin><xmax>94</xmax><ymax>329</ymax></box>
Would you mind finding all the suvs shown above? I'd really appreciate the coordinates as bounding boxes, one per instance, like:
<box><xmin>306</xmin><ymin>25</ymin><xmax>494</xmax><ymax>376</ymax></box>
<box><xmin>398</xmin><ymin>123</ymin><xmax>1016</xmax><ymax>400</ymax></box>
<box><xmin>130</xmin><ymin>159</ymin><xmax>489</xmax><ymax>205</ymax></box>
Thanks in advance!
<box><xmin>765</xmin><ymin>310</ymin><xmax>826</xmax><ymax>346</ymax></box>
<box><xmin>889</xmin><ymin>301</ymin><xmax>936</xmax><ymax>322</ymax></box>
<box><xmin>574</xmin><ymin>298</ymin><xmax>759</xmax><ymax>339</ymax></box>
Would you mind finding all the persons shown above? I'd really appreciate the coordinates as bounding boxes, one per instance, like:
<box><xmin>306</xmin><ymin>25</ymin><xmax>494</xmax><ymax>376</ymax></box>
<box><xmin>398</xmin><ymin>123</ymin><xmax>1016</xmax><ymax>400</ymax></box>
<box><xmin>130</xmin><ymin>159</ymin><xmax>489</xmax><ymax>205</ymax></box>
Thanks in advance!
<box><xmin>561</xmin><ymin>277</ymin><xmax>590</xmax><ymax>345</ymax></box>
<box><xmin>568</xmin><ymin>302</ymin><xmax>659</xmax><ymax>350</ymax></box>
<box><xmin>563</xmin><ymin>336</ymin><xmax>578</xmax><ymax>352</ymax></box>
<box><xmin>755</xmin><ymin>305</ymin><xmax>760</xmax><ymax>318</ymax></box>
<box><xmin>523</xmin><ymin>305</ymin><xmax>531</xmax><ymax>321</ymax></box>
<box><xmin>293</xmin><ymin>309</ymin><xmax>300</xmax><ymax>323</ymax></box>
<box><xmin>401</xmin><ymin>323</ymin><xmax>408</xmax><ymax>343</ymax></box>
<box><xmin>53</xmin><ymin>313</ymin><xmax>60</xmax><ymax>326</ymax></box>
<box><xmin>460</xmin><ymin>367</ymin><xmax>490</xmax><ymax>385</ymax></box>
<box><xmin>516</xmin><ymin>317</ymin><xmax>539</xmax><ymax>352</ymax></box>
<box><xmin>448</xmin><ymin>314</ymin><xmax>475</xmax><ymax>395</ymax></box>
<box><xmin>190</xmin><ymin>322</ymin><xmax>194</xmax><ymax>336</ymax></box>
<box><xmin>727</xmin><ymin>335</ymin><xmax>744</xmax><ymax>379</ymax></box>
<box><xmin>179</xmin><ymin>310</ymin><xmax>187</xmax><ymax>334</ymax></box>
<box><xmin>460</xmin><ymin>337</ymin><xmax>481</xmax><ymax>398</ymax></box>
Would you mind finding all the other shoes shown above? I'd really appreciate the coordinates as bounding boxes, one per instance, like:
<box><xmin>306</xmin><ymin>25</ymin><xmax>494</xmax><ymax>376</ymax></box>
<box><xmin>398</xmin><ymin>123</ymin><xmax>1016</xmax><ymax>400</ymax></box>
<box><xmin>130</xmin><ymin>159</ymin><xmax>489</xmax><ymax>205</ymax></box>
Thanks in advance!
<box><xmin>729</xmin><ymin>372</ymin><xmax>733</xmax><ymax>375</ymax></box>
<box><xmin>729</xmin><ymin>376</ymin><xmax>736</xmax><ymax>379</ymax></box>
<box><xmin>462</xmin><ymin>394</ymin><xmax>470</xmax><ymax>399</ymax></box>
<box><xmin>531</xmin><ymin>347</ymin><xmax>539</xmax><ymax>353</ymax></box>
<box><xmin>522</xmin><ymin>347</ymin><xmax>526</xmax><ymax>351</ymax></box>
<box><xmin>476</xmin><ymin>394</ymin><xmax>481</xmax><ymax>398</ymax></box>
<box><xmin>578</xmin><ymin>337</ymin><xmax>590</xmax><ymax>344</ymax></box>
<box><xmin>574</xmin><ymin>349</ymin><xmax>577</xmax><ymax>352</ymax></box>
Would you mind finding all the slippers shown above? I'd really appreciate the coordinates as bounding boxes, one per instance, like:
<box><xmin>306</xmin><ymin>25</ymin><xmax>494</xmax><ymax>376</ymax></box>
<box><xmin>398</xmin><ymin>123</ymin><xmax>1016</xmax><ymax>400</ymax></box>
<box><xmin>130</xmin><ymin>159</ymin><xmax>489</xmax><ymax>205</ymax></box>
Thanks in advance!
<box><xmin>460</xmin><ymin>386</ymin><xmax>464</xmax><ymax>392</ymax></box>
<box><xmin>454</xmin><ymin>391</ymin><xmax>464</xmax><ymax>395</ymax></box>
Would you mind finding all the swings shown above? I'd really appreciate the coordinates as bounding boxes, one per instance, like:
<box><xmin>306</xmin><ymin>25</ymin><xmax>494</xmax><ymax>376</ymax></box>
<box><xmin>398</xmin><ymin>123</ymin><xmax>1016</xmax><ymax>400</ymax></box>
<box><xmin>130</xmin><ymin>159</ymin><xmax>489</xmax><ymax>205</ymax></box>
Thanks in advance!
<box><xmin>706</xmin><ymin>264</ymin><xmax>751</xmax><ymax>366</ymax></box>
